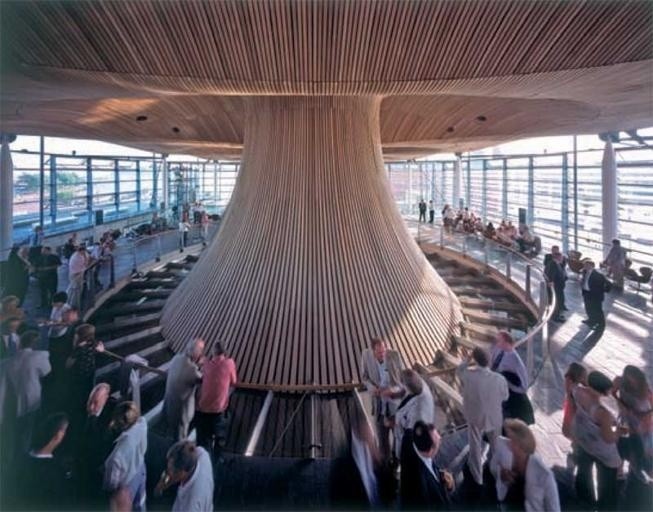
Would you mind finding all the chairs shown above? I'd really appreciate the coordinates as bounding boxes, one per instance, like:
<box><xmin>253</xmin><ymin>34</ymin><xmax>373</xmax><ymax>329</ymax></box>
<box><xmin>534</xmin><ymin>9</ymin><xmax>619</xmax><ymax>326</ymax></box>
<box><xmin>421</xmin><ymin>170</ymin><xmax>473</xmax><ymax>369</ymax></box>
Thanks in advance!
<box><xmin>568</xmin><ymin>250</ymin><xmax>591</xmax><ymax>280</ymax></box>
<box><xmin>631</xmin><ymin>266</ymin><xmax>652</xmax><ymax>290</ymax></box>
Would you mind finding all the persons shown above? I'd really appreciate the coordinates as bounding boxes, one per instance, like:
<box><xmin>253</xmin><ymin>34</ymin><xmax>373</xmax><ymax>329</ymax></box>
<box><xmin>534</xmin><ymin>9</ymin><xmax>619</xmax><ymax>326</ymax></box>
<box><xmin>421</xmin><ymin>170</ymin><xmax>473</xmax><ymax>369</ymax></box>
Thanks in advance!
<box><xmin>104</xmin><ymin>402</ymin><xmax>149</xmax><ymax>512</ymax></box>
<box><xmin>85</xmin><ymin>383</ymin><xmax>116</xmax><ymax>434</ymax></box>
<box><xmin>0</xmin><ymin>331</ymin><xmax>52</xmax><ymax>464</ymax></box>
<box><xmin>156</xmin><ymin>441</ymin><xmax>214</xmax><ymax>512</ymax></box>
<box><xmin>69</xmin><ymin>325</ymin><xmax>104</xmax><ymax>388</ymax></box>
<box><xmin>0</xmin><ymin>244</ymin><xmax>32</xmax><ymax>306</ymax></box>
<box><xmin>183</xmin><ymin>202</ymin><xmax>191</xmax><ymax>223</ymax></box>
<box><xmin>197</xmin><ymin>340</ymin><xmax>238</xmax><ymax>432</ymax></box>
<box><xmin>67</xmin><ymin>245</ymin><xmax>89</xmax><ymax>309</ymax></box>
<box><xmin>91</xmin><ymin>229</ymin><xmax>121</xmax><ymax>257</ymax></box>
<box><xmin>193</xmin><ymin>201</ymin><xmax>206</xmax><ymax>224</ymax></box>
<box><xmin>28</xmin><ymin>225</ymin><xmax>46</xmax><ymax>248</ymax></box>
<box><xmin>561</xmin><ymin>363</ymin><xmax>587</xmax><ymax>440</ymax></box>
<box><xmin>360</xmin><ymin>338</ymin><xmax>403</xmax><ymax>459</ymax></box>
<box><xmin>545</xmin><ymin>253</ymin><xmax>566</xmax><ymax>323</ymax></box>
<box><xmin>162</xmin><ymin>338</ymin><xmax>205</xmax><ymax>458</ymax></box>
<box><xmin>612</xmin><ymin>366</ymin><xmax>653</xmax><ymax>480</ymax></box>
<box><xmin>402</xmin><ymin>419</ymin><xmax>455</xmax><ymax>512</ymax></box>
<box><xmin>456</xmin><ymin>347</ymin><xmax>510</xmax><ymax>486</ymax></box>
<box><xmin>599</xmin><ymin>239</ymin><xmax>633</xmax><ymax>278</ymax></box>
<box><xmin>490</xmin><ymin>418</ymin><xmax>561</xmax><ymax>512</ymax></box>
<box><xmin>150</xmin><ymin>210</ymin><xmax>160</xmax><ymax>230</ymax></box>
<box><xmin>392</xmin><ymin>369</ymin><xmax>436</xmax><ymax>461</ymax></box>
<box><xmin>441</xmin><ymin>201</ymin><xmax>542</xmax><ymax>259</ymax></box>
<box><xmin>416</xmin><ymin>197</ymin><xmax>425</xmax><ymax>224</ymax></box>
<box><xmin>15</xmin><ymin>412</ymin><xmax>80</xmax><ymax>512</ymax></box>
<box><xmin>176</xmin><ymin>218</ymin><xmax>192</xmax><ymax>247</ymax></box>
<box><xmin>428</xmin><ymin>198</ymin><xmax>436</xmax><ymax>223</ymax></box>
<box><xmin>490</xmin><ymin>330</ymin><xmax>528</xmax><ymax>427</ymax></box>
<box><xmin>0</xmin><ymin>296</ymin><xmax>38</xmax><ymax>332</ymax></box>
<box><xmin>198</xmin><ymin>210</ymin><xmax>210</xmax><ymax>244</ymax></box>
<box><xmin>544</xmin><ymin>246</ymin><xmax>567</xmax><ymax>306</ymax></box>
<box><xmin>66</xmin><ymin>231</ymin><xmax>83</xmax><ymax>256</ymax></box>
<box><xmin>580</xmin><ymin>258</ymin><xmax>612</xmax><ymax>333</ymax></box>
<box><xmin>572</xmin><ymin>372</ymin><xmax>627</xmax><ymax>512</ymax></box>
<box><xmin>168</xmin><ymin>205</ymin><xmax>180</xmax><ymax>229</ymax></box>
<box><xmin>44</xmin><ymin>291</ymin><xmax>75</xmax><ymax>337</ymax></box>
<box><xmin>158</xmin><ymin>202</ymin><xmax>170</xmax><ymax>229</ymax></box>
<box><xmin>33</xmin><ymin>245</ymin><xmax>61</xmax><ymax>311</ymax></box>
